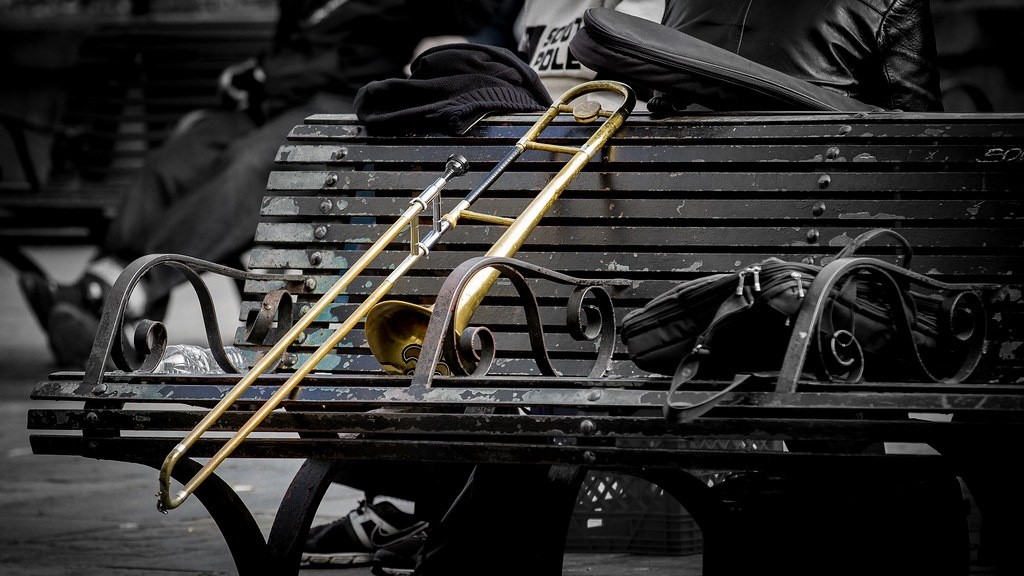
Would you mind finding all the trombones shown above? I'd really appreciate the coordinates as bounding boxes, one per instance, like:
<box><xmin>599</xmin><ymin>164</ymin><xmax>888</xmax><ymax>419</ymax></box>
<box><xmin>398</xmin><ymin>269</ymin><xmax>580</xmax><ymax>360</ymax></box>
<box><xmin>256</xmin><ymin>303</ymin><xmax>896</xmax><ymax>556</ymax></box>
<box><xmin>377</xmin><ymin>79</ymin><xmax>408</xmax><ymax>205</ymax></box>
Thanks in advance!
<box><xmin>154</xmin><ymin>76</ymin><xmax>639</xmax><ymax>512</ymax></box>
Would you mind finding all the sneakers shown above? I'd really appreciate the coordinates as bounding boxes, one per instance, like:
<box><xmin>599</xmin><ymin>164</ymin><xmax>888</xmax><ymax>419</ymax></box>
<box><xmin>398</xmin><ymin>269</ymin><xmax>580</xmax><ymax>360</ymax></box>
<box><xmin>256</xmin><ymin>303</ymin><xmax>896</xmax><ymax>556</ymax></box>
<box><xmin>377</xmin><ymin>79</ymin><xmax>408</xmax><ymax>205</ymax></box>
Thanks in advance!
<box><xmin>299</xmin><ymin>501</ymin><xmax>414</xmax><ymax>565</ymax></box>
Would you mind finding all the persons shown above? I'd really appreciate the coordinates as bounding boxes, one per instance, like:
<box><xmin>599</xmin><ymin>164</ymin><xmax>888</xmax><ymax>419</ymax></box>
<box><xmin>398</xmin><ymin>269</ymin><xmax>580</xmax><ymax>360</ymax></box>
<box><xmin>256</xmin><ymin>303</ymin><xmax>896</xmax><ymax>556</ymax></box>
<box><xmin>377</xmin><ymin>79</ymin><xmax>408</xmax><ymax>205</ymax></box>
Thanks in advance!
<box><xmin>17</xmin><ymin>0</ymin><xmax>411</xmax><ymax>366</ymax></box>
<box><xmin>301</xmin><ymin>0</ymin><xmax>939</xmax><ymax>576</ymax></box>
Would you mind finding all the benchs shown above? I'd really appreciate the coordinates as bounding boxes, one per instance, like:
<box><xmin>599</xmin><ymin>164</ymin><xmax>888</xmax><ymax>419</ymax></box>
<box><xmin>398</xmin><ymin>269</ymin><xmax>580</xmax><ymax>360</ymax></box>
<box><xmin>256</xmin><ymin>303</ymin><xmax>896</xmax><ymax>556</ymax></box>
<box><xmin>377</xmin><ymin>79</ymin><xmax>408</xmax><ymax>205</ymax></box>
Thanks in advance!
<box><xmin>0</xmin><ymin>12</ymin><xmax>277</xmax><ymax>327</ymax></box>
<box><xmin>27</xmin><ymin>109</ymin><xmax>1024</xmax><ymax>576</ymax></box>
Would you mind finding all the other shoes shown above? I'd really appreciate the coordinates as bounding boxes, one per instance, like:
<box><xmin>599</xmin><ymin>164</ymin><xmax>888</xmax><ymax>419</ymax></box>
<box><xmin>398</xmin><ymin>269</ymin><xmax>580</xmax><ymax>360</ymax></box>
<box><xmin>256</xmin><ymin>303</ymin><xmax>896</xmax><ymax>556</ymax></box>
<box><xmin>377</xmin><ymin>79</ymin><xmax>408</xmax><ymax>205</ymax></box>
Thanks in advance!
<box><xmin>21</xmin><ymin>272</ymin><xmax>98</xmax><ymax>369</ymax></box>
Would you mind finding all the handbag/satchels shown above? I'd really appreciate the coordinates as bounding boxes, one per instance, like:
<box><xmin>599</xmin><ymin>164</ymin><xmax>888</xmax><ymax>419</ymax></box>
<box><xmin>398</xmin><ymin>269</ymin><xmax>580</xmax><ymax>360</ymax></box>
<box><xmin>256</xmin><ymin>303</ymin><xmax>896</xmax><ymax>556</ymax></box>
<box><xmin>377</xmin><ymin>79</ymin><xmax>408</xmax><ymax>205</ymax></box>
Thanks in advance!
<box><xmin>619</xmin><ymin>227</ymin><xmax>1002</xmax><ymax>424</ymax></box>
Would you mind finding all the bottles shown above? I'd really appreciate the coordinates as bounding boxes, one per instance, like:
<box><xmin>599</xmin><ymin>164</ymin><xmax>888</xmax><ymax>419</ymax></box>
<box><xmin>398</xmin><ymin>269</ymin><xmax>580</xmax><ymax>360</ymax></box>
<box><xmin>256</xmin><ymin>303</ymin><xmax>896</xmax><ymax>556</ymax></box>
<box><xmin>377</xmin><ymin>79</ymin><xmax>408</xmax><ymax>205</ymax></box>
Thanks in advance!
<box><xmin>144</xmin><ymin>345</ymin><xmax>249</xmax><ymax>375</ymax></box>
